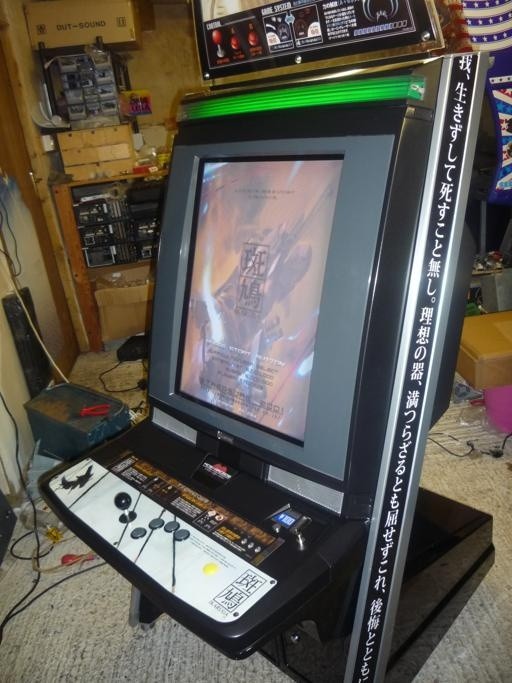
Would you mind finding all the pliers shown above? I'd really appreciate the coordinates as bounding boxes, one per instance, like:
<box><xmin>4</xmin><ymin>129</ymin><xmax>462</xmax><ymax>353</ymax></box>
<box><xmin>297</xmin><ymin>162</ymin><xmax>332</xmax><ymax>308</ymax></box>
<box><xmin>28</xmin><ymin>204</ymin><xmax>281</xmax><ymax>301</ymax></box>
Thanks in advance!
<box><xmin>70</xmin><ymin>404</ymin><xmax>111</xmax><ymax>418</ymax></box>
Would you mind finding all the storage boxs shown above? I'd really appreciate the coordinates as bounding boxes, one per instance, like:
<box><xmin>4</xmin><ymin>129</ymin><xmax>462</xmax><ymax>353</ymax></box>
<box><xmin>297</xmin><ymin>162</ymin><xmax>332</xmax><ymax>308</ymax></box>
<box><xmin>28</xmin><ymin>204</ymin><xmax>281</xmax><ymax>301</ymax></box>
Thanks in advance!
<box><xmin>23</xmin><ymin>0</ymin><xmax>143</xmax><ymax>53</ymax></box>
<box><xmin>457</xmin><ymin>309</ymin><xmax>511</xmax><ymax>390</ymax></box>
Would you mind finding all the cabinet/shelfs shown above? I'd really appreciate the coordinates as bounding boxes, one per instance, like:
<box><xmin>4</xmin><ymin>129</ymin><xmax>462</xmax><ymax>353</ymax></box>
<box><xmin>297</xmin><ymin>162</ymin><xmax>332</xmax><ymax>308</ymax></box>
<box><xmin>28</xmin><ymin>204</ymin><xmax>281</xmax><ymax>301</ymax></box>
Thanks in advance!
<box><xmin>51</xmin><ymin>168</ymin><xmax>171</xmax><ymax>354</ymax></box>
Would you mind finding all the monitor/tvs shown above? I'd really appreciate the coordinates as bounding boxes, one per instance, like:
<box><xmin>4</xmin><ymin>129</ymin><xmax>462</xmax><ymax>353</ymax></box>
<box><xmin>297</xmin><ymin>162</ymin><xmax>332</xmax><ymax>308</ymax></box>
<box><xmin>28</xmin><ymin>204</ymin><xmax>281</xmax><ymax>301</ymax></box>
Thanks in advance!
<box><xmin>148</xmin><ymin>134</ymin><xmax>396</xmax><ymax>482</ymax></box>
